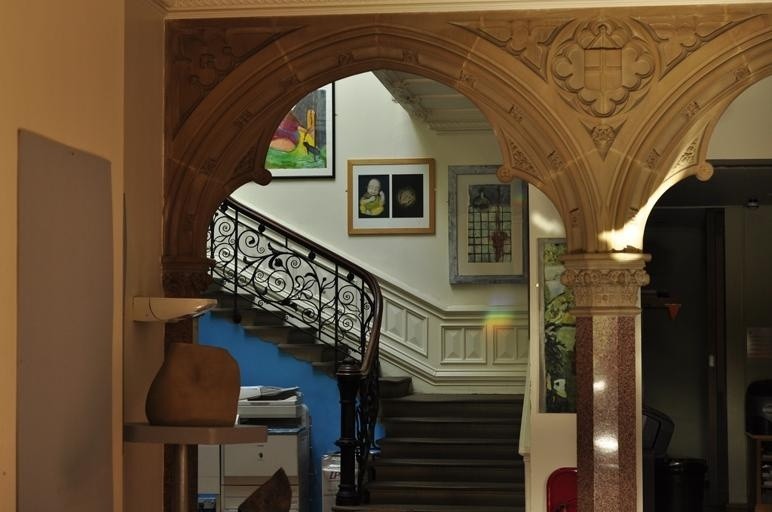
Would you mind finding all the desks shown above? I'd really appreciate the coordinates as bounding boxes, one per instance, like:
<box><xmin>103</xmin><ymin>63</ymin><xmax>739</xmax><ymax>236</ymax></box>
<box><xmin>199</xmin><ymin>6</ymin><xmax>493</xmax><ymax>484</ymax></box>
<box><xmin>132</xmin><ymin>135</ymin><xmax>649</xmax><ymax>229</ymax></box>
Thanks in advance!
<box><xmin>120</xmin><ymin>424</ymin><xmax>266</xmax><ymax>510</ymax></box>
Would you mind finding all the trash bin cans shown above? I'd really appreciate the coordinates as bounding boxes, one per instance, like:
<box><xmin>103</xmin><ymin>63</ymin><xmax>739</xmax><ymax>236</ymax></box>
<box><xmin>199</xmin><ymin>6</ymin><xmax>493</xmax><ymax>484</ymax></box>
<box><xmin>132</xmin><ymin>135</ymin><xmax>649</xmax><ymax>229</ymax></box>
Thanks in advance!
<box><xmin>655</xmin><ymin>457</ymin><xmax>706</xmax><ymax>512</ymax></box>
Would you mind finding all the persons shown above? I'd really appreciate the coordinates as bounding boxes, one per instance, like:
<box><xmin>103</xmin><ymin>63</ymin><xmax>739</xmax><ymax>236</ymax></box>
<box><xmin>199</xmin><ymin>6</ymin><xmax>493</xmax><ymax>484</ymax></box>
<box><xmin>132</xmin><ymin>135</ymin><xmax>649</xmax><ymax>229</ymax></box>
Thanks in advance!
<box><xmin>360</xmin><ymin>177</ymin><xmax>386</xmax><ymax>216</ymax></box>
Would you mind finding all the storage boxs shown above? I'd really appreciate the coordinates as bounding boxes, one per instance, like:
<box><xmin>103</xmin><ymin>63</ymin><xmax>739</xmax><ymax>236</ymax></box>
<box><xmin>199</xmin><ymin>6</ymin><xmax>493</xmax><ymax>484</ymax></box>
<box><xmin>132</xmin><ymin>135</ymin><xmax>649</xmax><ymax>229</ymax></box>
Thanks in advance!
<box><xmin>321</xmin><ymin>452</ymin><xmax>380</xmax><ymax>510</ymax></box>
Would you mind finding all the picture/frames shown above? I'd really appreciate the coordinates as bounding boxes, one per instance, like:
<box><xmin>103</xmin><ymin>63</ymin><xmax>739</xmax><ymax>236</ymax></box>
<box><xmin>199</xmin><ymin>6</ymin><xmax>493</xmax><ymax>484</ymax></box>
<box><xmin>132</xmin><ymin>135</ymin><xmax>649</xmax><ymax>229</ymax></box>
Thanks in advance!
<box><xmin>346</xmin><ymin>160</ymin><xmax>435</xmax><ymax>237</ymax></box>
<box><xmin>447</xmin><ymin>165</ymin><xmax>529</xmax><ymax>287</ymax></box>
<box><xmin>259</xmin><ymin>82</ymin><xmax>335</xmax><ymax>179</ymax></box>
<box><xmin>537</xmin><ymin>237</ymin><xmax>592</xmax><ymax>415</ymax></box>
